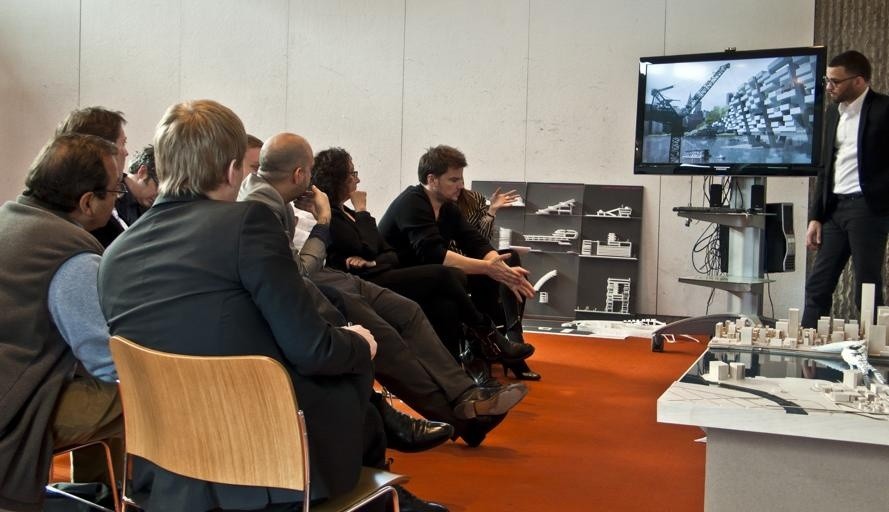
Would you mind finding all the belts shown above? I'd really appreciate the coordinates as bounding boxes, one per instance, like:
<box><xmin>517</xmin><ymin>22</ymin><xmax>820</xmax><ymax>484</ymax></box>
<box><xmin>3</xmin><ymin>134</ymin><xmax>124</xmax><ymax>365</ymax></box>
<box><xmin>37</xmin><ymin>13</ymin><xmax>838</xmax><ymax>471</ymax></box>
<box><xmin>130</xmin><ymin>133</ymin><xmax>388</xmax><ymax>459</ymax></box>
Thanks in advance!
<box><xmin>833</xmin><ymin>192</ymin><xmax>865</xmax><ymax>201</ymax></box>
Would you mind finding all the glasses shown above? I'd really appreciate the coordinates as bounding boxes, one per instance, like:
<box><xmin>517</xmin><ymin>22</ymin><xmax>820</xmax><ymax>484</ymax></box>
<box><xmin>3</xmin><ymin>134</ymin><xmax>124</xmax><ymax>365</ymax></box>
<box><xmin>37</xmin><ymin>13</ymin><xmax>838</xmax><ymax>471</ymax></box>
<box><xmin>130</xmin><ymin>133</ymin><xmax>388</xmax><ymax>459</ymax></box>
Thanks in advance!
<box><xmin>104</xmin><ymin>183</ymin><xmax>130</xmax><ymax>200</ymax></box>
<box><xmin>822</xmin><ymin>75</ymin><xmax>858</xmax><ymax>87</ymax></box>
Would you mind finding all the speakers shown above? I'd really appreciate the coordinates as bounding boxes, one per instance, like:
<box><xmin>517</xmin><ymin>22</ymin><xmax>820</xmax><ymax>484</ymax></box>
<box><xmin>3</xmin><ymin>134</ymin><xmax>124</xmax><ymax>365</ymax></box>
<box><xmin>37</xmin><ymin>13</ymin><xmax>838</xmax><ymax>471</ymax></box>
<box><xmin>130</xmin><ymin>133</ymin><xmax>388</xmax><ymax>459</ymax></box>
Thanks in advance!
<box><xmin>711</xmin><ymin>184</ymin><xmax>722</xmax><ymax>204</ymax></box>
<box><xmin>751</xmin><ymin>185</ymin><xmax>766</xmax><ymax>212</ymax></box>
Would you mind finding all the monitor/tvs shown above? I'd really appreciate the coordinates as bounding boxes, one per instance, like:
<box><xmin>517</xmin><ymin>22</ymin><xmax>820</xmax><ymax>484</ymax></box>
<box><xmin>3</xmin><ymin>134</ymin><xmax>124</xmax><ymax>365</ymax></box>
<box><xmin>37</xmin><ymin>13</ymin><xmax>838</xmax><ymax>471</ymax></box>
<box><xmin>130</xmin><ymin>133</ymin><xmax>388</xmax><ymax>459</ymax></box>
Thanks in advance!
<box><xmin>634</xmin><ymin>45</ymin><xmax>828</xmax><ymax>176</ymax></box>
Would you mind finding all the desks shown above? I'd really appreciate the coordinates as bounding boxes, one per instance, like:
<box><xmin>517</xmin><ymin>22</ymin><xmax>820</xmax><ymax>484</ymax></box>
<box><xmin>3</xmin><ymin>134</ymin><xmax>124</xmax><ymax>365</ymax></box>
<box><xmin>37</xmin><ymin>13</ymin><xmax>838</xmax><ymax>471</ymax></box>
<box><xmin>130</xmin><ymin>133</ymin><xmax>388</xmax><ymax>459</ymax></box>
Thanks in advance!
<box><xmin>653</xmin><ymin>340</ymin><xmax>888</xmax><ymax>512</ymax></box>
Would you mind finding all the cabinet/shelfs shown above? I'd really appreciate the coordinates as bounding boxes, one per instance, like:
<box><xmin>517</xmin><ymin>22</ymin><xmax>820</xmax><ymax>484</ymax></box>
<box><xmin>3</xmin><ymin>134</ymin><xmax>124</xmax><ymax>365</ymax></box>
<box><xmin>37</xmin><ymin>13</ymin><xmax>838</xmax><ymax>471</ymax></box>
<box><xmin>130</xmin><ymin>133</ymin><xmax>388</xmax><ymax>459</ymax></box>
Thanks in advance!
<box><xmin>482</xmin><ymin>202</ymin><xmax>640</xmax><ymax>317</ymax></box>
<box><xmin>650</xmin><ymin>206</ymin><xmax>778</xmax><ymax>355</ymax></box>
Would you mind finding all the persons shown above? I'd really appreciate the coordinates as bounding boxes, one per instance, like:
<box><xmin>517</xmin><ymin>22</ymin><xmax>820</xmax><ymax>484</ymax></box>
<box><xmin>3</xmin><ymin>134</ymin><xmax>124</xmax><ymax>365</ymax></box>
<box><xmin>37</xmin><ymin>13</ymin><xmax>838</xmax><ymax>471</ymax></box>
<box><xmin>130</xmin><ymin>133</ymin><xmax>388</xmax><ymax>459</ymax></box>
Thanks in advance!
<box><xmin>800</xmin><ymin>50</ymin><xmax>889</xmax><ymax>328</ymax></box>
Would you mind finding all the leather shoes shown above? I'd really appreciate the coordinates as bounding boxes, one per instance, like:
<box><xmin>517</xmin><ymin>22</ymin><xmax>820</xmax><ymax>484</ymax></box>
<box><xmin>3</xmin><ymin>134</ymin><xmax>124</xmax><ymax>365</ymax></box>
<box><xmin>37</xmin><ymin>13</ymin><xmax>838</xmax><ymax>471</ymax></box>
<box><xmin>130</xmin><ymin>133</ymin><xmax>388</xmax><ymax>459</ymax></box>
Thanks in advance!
<box><xmin>472</xmin><ymin>330</ymin><xmax>537</xmax><ymax>366</ymax></box>
<box><xmin>452</xmin><ymin>383</ymin><xmax>529</xmax><ymax>420</ymax></box>
<box><xmin>461</xmin><ymin>412</ymin><xmax>507</xmax><ymax>448</ymax></box>
<box><xmin>365</xmin><ymin>464</ymin><xmax>450</xmax><ymax>512</ymax></box>
<box><xmin>371</xmin><ymin>392</ymin><xmax>456</xmax><ymax>454</ymax></box>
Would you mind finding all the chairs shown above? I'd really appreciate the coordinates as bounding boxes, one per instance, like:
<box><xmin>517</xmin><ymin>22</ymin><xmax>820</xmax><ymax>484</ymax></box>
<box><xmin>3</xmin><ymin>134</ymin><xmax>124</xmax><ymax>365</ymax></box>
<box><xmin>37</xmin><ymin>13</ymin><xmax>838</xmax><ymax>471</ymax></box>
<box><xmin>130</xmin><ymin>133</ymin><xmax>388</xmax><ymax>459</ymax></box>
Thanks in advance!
<box><xmin>106</xmin><ymin>333</ymin><xmax>411</xmax><ymax>510</ymax></box>
<box><xmin>51</xmin><ymin>441</ymin><xmax>125</xmax><ymax>512</ymax></box>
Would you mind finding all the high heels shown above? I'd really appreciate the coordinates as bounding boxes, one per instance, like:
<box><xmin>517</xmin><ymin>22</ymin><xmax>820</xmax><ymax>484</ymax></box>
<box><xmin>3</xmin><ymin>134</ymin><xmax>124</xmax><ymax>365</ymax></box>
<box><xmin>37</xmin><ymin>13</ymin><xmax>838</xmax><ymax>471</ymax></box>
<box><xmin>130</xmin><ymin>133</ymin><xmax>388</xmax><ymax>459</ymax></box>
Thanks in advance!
<box><xmin>503</xmin><ymin>358</ymin><xmax>541</xmax><ymax>380</ymax></box>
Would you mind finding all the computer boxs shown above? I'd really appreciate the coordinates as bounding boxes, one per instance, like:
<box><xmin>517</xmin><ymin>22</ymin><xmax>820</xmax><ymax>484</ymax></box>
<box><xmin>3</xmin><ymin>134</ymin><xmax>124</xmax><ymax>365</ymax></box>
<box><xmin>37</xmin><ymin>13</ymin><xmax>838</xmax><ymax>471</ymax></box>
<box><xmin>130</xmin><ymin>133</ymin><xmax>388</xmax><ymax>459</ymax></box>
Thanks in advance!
<box><xmin>719</xmin><ymin>203</ymin><xmax>796</xmax><ymax>273</ymax></box>
